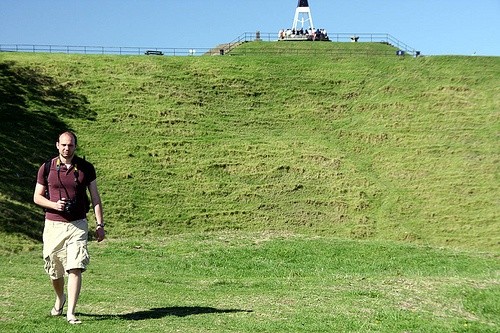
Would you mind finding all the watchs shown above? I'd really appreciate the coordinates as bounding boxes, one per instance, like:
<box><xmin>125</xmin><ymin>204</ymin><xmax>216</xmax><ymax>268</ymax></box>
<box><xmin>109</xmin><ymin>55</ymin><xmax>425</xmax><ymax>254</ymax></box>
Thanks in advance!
<box><xmin>97</xmin><ymin>223</ymin><xmax>104</xmax><ymax>227</ymax></box>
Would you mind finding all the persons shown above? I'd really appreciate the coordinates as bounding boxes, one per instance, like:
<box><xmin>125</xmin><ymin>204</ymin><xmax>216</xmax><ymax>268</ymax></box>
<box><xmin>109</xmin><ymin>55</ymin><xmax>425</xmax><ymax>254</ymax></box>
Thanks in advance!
<box><xmin>277</xmin><ymin>27</ymin><xmax>327</xmax><ymax>41</ymax></box>
<box><xmin>34</xmin><ymin>131</ymin><xmax>106</xmax><ymax>324</ymax></box>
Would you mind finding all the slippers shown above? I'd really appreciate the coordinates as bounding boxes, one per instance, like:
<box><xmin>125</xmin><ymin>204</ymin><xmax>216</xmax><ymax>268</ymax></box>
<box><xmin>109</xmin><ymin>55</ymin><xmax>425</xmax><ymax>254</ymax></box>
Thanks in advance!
<box><xmin>68</xmin><ymin>319</ymin><xmax>82</xmax><ymax>324</ymax></box>
<box><xmin>50</xmin><ymin>299</ymin><xmax>66</xmax><ymax>316</ymax></box>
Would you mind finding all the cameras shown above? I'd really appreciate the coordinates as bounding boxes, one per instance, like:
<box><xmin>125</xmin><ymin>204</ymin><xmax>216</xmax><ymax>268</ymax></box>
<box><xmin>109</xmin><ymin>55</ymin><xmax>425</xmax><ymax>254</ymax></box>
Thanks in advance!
<box><xmin>64</xmin><ymin>202</ymin><xmax>72</xmax><ymax>214</ymax></box>
